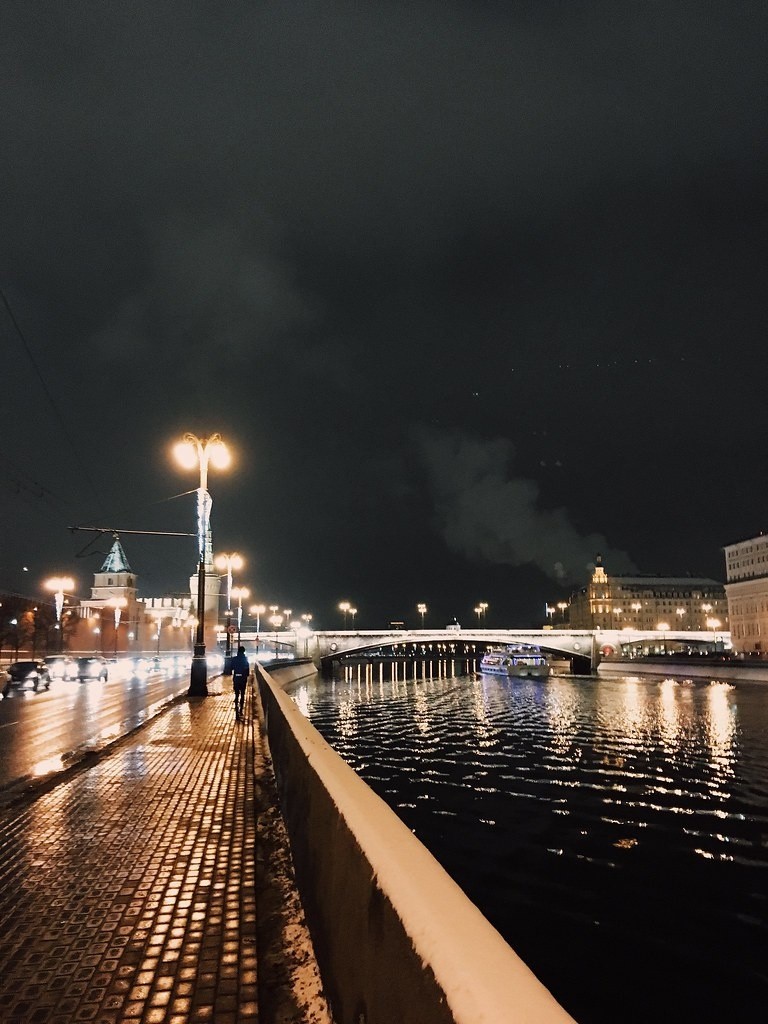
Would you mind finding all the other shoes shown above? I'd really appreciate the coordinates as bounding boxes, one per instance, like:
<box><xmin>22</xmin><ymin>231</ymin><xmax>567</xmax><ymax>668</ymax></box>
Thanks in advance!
<box><xmin>240</xmin><ymin>699</ymin><xmax>244</xmax><ymax>704</ymax></box>
<box><xmin>234</xmin><ymin>698</ymin><xmax>238</xmax><ymax>702</ymax></box>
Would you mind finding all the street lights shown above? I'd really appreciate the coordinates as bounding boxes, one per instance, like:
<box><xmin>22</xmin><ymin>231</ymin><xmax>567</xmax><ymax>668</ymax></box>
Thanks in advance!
<box><xmin>107</xmin><ymin>594</ymin><xmax>128</xmax><ymax>658</ymax></box>
<box><xmin>270</xmin><ymin>616</ymin><xmax>284</xmax><ymax>661</ymax></box>
<box><xmin>216</xmin><ymin>549</ymin><xmax>244</xmax><ymax>675</ymax></box>
<box><xmin>250</xmin><ymin>603</ymin><xmax>313</xmax><ymax>658</ymax></box>
<box><xmin>171</xmin><ymin>432</ymin><xmax>236</xmax><ymax>698</ymax></box>
<box><xmin>419</xmin><ymin>604</ymin><xmax>427</xmax><ymax>630</ymax></box>
<box><xmin>340</xmin><ymin>601</ymin><xmax>351</xmax><ymax>631</ymax></box>
<box><xmin>350</xmin><ymin>607</ymin><xmax>357</xmax><ymax>631</ymax></box>
<box><xmin>49</xmin><ymin>573</ymin><xmax>77</xmax><ymax>653</ymax></box>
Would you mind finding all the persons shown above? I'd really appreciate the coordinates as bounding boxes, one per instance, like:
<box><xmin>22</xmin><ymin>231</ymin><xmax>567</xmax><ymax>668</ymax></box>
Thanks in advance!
<box><xmin>231</xmin><ymin>646</ymin><xmax>249</xmax><ymax>719</ymax></box>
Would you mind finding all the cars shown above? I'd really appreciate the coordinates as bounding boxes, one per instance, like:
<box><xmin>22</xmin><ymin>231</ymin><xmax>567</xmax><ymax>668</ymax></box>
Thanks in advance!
<box><xmin>5</xmin><ymin>660</ymin><xmax>52</xmax><ymax>695</ymax></box>
<box><xmin>43</xmin><ymin>646</ymin><xmax>294</xmax><ymax>685</ymax></box>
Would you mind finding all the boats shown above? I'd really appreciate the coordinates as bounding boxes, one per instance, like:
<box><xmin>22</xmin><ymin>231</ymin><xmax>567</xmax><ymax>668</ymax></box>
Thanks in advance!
<box><xmin>479</xmin><ymin>642</ymin><xmax>554</xmax><ymax>679</ymax></box>
